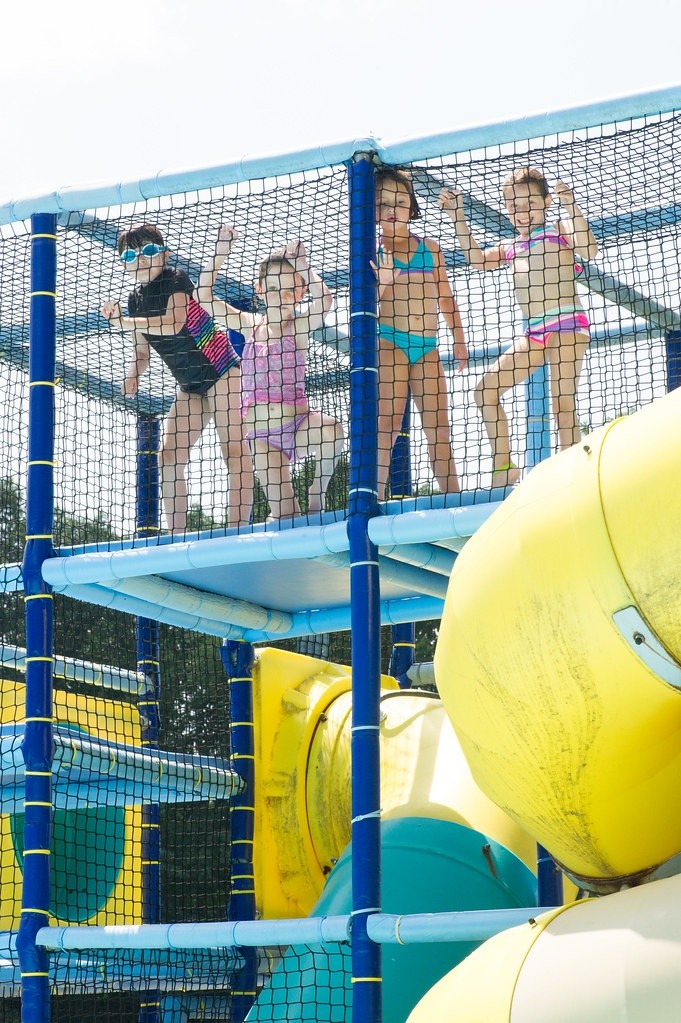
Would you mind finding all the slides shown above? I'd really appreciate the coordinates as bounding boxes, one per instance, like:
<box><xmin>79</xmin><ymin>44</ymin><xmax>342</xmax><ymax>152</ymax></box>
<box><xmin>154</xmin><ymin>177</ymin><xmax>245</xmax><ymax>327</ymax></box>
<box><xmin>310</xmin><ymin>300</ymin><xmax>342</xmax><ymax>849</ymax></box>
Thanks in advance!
<box><xmin>397</xmin><ymin>376</ymin><xmax>681</xmax><ymax>1023</ymax></box>
<box><xmin>241</xmin><ymin>684</ymin><xmax>538</xmax><ymax>1023</ymax></box>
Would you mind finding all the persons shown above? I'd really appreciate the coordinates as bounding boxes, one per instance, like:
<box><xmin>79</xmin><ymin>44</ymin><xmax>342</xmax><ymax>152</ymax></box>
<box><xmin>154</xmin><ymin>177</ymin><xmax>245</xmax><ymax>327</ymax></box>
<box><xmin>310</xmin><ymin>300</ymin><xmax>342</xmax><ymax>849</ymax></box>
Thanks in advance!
<box><xmin>370</xmin><ymin>170</ymin><xmax>467</xmax><ymax>500</ymax></box>
<box><xmin>101</xmin><ymin>224</ymin><xmax>254</xmax><ymax>534</ymax></box>
<box><xmin>192</xmin><ymin>226</ymin><xmax>343</xmax><ymax>521</ymax></box>
<box><xmin>438</xmin><ymin>167</ymin><xmax>598</xmax><ymax>486</ymax></box>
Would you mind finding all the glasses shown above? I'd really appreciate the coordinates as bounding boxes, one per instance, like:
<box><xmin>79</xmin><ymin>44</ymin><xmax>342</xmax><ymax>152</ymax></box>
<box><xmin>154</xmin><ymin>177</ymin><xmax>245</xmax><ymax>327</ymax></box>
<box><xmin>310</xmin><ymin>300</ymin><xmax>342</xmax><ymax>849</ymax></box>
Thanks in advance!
<box><xmin>119</xmin><ymin>244</ymin><xmax>166</xmax><ymax>263</ymax></box>
